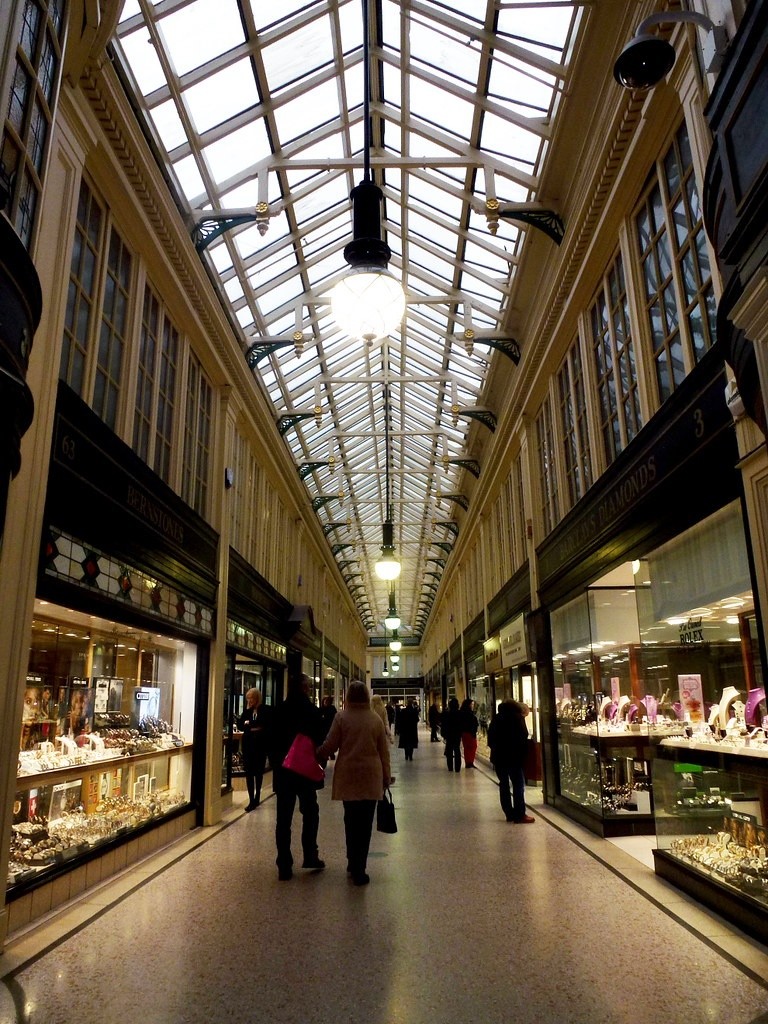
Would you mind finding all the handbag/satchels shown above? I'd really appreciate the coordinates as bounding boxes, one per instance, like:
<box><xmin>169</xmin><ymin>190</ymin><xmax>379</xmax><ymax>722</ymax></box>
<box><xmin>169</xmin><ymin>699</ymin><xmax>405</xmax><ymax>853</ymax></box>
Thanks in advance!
<box><xmin>282</xmin><ymin>734</ymin><xmax>325</xmax><ymax>781</ymax></box>
<box><xmin>377</xmin><ymin>789</ymin><xmax>398</xmax><ymax>833</ymax></box>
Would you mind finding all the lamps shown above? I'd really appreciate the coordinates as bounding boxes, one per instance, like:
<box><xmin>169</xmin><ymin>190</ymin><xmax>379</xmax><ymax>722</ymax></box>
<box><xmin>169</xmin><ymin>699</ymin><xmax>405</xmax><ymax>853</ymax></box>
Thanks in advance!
<box><xmin>373</xmin><ymin>381</ymin><xmax>403</xmax><ymax>583</ymax></box>
<box><xmin>611</xmin><ymin>8</ymin><xmax>728</xmax><ymax>93</ymax></box>
<box><xmin>381</xmin><ymin>626</ymin><xmax>390</xmax><ymax>677</ymax></box>
<box><xmin>390</xmin><ymin>651</ymin><xmax>400</xmax><ymax>662</ymax></box>
<box><xmin>391</xmin><ymin>663</ymin><xmax>400</xmax><ymax>672</ymax></box>
<box><xmin>389</xmin><ymin>629</ymin><xmax>402</xmax><ymax>651</ymax></box>
<box><xmin>328</xmin><ymin>0</ymin><xmax>409</xmax><ymax>345</ymax></box>
<box><xmin>384</xmin><ymin>588</ymin><xmax>401</xmax><ymax>630</ymax></box>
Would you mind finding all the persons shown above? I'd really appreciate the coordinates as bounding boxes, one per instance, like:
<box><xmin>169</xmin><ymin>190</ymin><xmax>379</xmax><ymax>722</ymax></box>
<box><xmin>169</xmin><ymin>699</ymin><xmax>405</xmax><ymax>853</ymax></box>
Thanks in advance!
<box><xmin>236</xmin><ymin>688</ymin><xmax>271</xmax><ymax>812</ymax></box>
<box><xmin>441</xmin><ymin>698</ymin><xmax>479</xmax><ymax>772</ymax></box>
<box><xmin>317</xmin><ymin>681</ymin><xmax>391</xmax><ymax>884</ymax></box>
<box><xmin>386</xmin><ymin>698</ymin><xmax>421</xmax><ymax>761</ymax></box>
<box><xmin>428</xmin><ymin>702</ymin><xmax>441</xmax><ymax>742</ymax></box>
<box><xmin>319</xmin><ymin>697</ymin><xmax>338</xmax><ymax>760</ymax></box>
<box><xmin>486</xmin><ymin>698</ymin><xmax>535</xmax><ymax>823</ymax></box>
<box><xmin>268</xmin><ymin>674</ymin><xmax>325</xmax><ymax>881</ymax></box>
<box><xmin>371</xmin><ymin>694</ymin><xmax>394</xmax><ymax>744</ymax></box>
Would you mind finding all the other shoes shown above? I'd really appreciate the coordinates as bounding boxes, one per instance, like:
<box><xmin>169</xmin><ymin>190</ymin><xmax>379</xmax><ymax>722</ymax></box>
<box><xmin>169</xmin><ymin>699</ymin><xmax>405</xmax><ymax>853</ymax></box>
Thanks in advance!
<box><xmin>351</xmin><ymin>866</ymin><xmax>369</xmax><ymax>885</ymax></box>
<box><xmin>516</xmin><ymin>816</ymin><xmax>535</xmax><ymax>823</ymax></box>
<box><xmin>302</xmin><ymin>858</ymin><xmax>325</xmax><ymax>868</ymax></box>
<box><xmin>347</xmin><ymin>860</ymin><xmax>352</xmax><ymax>873</ymax></box>
<box><xmin>278</xmin><ymin>865</ymin><xmax>293</xmax><ymax>880</ymax></box>
<box><xmin>245</xmin><ymin>803</ymin><xmax>256</xmax><ymax>811</ymax></box>
<box><xmin>253</xmin><ymin>799</ymin><xmax>260</xmax><ymax>806</ymax></box>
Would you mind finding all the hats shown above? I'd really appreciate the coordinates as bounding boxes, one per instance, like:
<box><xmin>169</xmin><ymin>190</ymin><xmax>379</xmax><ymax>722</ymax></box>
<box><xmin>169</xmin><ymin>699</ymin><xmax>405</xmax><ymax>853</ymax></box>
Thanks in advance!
<box><xmin>290</xmin><ymin>672</ymin><xmax>306</xmax><ymax>689</ymax></box>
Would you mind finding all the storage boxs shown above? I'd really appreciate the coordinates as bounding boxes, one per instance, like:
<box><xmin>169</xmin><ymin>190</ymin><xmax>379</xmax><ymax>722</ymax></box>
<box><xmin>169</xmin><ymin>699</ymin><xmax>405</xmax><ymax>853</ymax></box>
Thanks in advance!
<box><xmin>7</xmin><ymin>746</ymin><xmax>194</xmax><ymax>898</ymax></box>
<box><xmin>557</xmin><ymin>732</ymin><xmax>650</xmax><ymax>818</ymax></box>
<box><xmin>647</xmin><ymin>747</ymin><xmax>768</xmax><ymax>911</ymax></box>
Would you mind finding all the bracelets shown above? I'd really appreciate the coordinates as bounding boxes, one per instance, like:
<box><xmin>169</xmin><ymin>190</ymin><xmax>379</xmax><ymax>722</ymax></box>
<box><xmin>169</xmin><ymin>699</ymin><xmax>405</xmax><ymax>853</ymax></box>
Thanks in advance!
<box><xmin>670</xmin><ymin>835</ymin><xmax>746</xmax><ymax>875</ymax></box>
